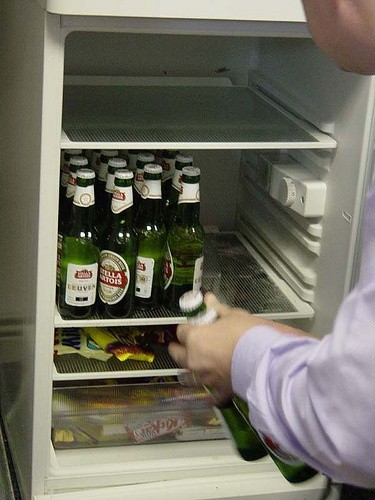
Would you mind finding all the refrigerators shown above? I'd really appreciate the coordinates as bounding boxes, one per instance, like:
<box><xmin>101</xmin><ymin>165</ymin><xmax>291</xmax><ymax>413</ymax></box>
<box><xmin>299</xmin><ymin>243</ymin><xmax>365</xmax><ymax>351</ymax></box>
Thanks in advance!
<box><xmin>0</xmin><ymin>0</ymin><xmax>375</xmax><ymax>500</ymax></box>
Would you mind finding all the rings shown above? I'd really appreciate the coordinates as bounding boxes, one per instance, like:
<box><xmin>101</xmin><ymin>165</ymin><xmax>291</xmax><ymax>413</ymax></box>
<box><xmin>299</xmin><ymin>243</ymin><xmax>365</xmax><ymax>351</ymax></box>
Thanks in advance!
<box><xmin>188</xmin><ymin>370</ymin><xmax>202</xmax><ymax>391</ymax></box>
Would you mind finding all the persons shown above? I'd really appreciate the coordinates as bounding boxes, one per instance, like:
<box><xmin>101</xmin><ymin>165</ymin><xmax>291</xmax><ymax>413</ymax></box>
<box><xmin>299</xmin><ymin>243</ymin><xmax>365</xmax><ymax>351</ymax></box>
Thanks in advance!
<box><xmin>165</xmin><ymin>0</ymin><xmax>375</xmax><ymax>500</ymax></box>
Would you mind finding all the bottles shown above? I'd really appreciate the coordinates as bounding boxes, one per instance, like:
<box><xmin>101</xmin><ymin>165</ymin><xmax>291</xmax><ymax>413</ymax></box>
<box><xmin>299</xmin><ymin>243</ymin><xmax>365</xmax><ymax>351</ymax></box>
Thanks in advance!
<box><xmin>180</xmin><ymin>290</ymin><xmax>267</xmax><ymax>462</ymax></box>
<box><xmin>92</xmin><ymin>150</ymin><xmax>102</xmax><ymax>169</ymax></box>
<box><xmin>172</xmin><ymin>154</ymin><xmax>193</xmax><ymax>204</ymax></box>
<box><xmin>166</xmin><ymin>166</ymin><xmax>205</xmax><ymax>314</ymax></box>
<box><xmin>99</xmin><ymin>169</ymin><xmax>135</xmax><ymax>319</ymax></box>
<box><xmin>105</xmin><ymin>158</ymin><xmax>128</xmax><ymax>210</ymax></box>
<box><xmin>160</xmin><ymin>149</ymin><xmax>176</xmax><ymax>182</ymax></box>
<box><xmin>98</xmin><ymin>150</ymin><xmax>119</xmax><ymax>182</ymax></box>
<box><xmin>56</xmin><ymin>156</ymin><xmax>89</xmax><ymax>307</ymax></box>
<box><xmin>133</xmin><ymin>153</ymin><xmax>155</xmax><ymax>195</ymax></box>
<box><xmin>61</xmin><ymin>149</ymin><xmax>82</xmax><ymax>187</ymax></box>
<box><xmin>127</xmin><ymin>149</ymin><xmax>139</xmax><ymax>171</ymax></box>
<box><xmin>59</xmin><ymin>168</ymin><xmax>99</xmax><ymax>319</ymax></box>
<box><xmin>135</xmin><ymin>163</ymin><xmax>167</xmax><ymax>310</ymax></box>
<box><xmin>203</xmin><ymin>306</ymin><xmax>319</xmax><ymax>484</ymax></box>
